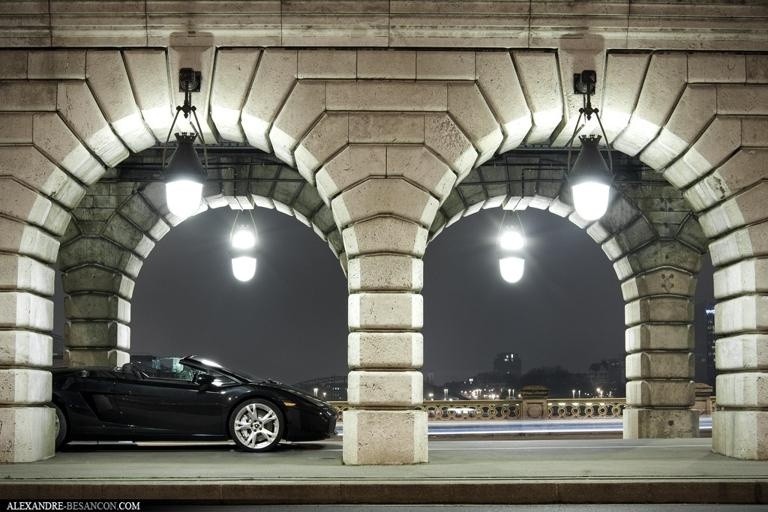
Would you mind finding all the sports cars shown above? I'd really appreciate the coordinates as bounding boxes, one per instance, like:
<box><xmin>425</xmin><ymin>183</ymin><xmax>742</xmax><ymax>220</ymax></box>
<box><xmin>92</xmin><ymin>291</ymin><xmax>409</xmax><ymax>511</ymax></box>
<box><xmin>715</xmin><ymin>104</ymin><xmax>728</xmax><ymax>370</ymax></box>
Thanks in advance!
<box><xmin>54</xmin><ymin>351</ymin><xmax>338</xmax><ymax>452</ymax></box>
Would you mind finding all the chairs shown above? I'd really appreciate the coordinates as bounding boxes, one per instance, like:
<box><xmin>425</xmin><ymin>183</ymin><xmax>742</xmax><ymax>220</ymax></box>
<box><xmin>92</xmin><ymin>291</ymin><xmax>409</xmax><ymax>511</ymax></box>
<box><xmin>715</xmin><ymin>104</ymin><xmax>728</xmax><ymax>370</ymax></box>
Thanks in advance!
<box><xmin>120</xmin><ymin>362</ymin><xmax>150</xmax><ymax>380</ymax></box>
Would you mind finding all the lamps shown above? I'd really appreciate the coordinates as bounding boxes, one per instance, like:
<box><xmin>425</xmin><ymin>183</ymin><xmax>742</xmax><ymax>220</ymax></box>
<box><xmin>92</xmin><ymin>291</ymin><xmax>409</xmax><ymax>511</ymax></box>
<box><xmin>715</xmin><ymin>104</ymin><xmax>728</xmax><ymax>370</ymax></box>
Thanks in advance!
<box><xmin>496</xmin><ymin>210</ymin><xmax>527</xmax><ymax>284</ymax></box>
<box><xmin>228</xmin><ymin>211</ymin><xmax>260</xmax><ymax>283</ymax></box>
<box><xmin>162</xmin><ymin>69</ymin><xmax>210</xmax><ymax>221</ymax></box>
<box><xmin>566</xmin><ymin>69</ymin><xmax>614</xmax><ymax>222</ymax></box>
